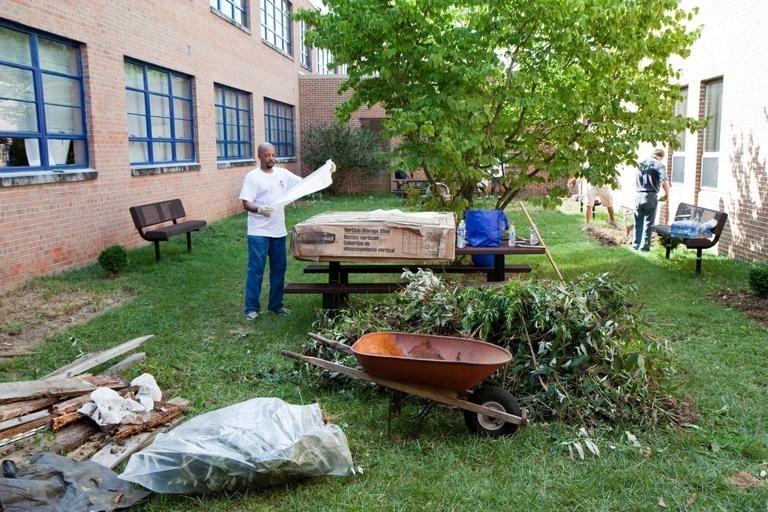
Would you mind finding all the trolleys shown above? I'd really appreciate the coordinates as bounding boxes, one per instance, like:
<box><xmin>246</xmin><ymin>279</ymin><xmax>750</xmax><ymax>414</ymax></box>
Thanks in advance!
<box><xmin>279</xmin><ymin>330</ymin><xmax>531</xmax><ymax>438</ymax></box>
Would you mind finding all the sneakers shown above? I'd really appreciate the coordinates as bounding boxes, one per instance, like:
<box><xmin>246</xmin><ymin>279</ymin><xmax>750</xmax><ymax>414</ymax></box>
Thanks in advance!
<box><xmin>607</xmin><ymin>220</ymin><xmax>622</xmax><ymax>231</ymax></box>
<box><xmin>246</xmin><ymin>310</ymin><xmax>259</xmax><ymax>321</ymax></box>
<box><xmin>268</xmin><ymin>307</ymin><xmax>292</xmax><ymax>315</ymax></box>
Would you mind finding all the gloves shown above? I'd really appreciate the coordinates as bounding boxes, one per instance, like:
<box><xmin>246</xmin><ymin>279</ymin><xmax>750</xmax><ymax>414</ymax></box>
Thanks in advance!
<box><xmin>257</xmin><ymin>205</ymin><xmax>273</xmax><ymax>218</ymax></box>
<box><xmin>325</xmin><ymin>159</ymin><xmax>336</xmax><ymax>173</ymax></box>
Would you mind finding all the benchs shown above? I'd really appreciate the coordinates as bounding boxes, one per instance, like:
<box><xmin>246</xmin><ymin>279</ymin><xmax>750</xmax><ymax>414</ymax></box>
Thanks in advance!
<box><xmin>653</xmin><ymin>202</ymin><xmax>727</xmax><ymax>275</ymax></box>
<box><xmin>130</xmin><ymin>199</ymin><xmax>206</xmax><ymax>261</ymax></box>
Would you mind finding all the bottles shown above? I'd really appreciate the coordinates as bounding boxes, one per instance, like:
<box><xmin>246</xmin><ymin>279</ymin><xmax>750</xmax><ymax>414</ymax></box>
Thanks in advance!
<box><xmin>508</xmin><ymin>224</ymin><xmax>516</xmax><ymax>247</ymax></box>
<box><xmin>456</xmin><ymin>220</ymin><xmax>466</xmax><ymax>249</ymax></box>
<box><xmin>529</xmin><ymin>227</ymin><xmax>535</xmax><ymax>245</ymax></box>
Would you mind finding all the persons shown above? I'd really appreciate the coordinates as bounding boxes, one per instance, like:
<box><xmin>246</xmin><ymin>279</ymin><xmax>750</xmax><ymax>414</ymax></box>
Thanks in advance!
<box><xmin>488</xmin><ymin>157</ymin><xmax>505</xmax><ymax>199</ymax></box>
<box><xmin>632</xmin><ymin>149</ymin><xmax>669</xmax><ymax>251</ymax></box>
<box><xmin>571</xmin><ymin>161</ymin><xmax>623</xmax><ymax>231</ymax></box>
<box><xmin>238</xmin><ymin>142</ymin><xmax>336</xmax><ymax>321</ymax></box>
<box><xmin>476</xmin><ymin>163</ymin><xmax>491</xmax><ymax>200</ymax></box>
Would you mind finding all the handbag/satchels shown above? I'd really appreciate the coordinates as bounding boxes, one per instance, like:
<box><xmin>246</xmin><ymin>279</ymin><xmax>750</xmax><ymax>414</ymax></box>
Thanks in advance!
<box><xmin>465</xmin><ymin>210</ymin><xmax>508</xmax><ymax>248</ymax></box>
